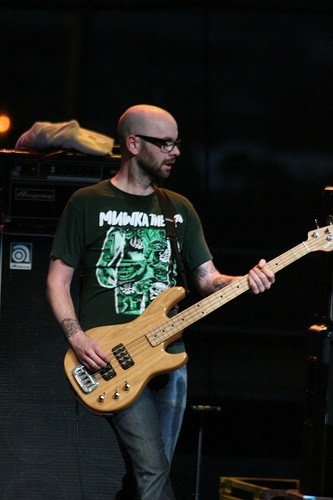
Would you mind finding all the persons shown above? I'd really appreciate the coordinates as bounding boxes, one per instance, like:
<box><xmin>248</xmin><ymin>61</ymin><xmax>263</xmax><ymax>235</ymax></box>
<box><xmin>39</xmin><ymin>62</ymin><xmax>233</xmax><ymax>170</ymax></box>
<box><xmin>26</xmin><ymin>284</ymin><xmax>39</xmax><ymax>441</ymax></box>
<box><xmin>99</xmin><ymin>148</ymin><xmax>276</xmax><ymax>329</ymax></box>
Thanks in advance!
<box><xmin>47</xmin><ymin>104</ymin><xmax>275</xmax><ymax>499</ymax></box>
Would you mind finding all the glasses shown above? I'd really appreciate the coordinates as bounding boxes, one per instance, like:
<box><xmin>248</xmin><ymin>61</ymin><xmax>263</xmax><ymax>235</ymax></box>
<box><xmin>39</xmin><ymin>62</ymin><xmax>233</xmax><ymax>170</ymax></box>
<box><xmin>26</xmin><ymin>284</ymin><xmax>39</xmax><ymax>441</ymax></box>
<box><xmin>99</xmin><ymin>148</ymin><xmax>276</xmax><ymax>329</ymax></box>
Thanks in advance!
<box><xmin>135</xmin><ymin>135</ymin><xmax>182</xmax><ymax>153</ymax></box>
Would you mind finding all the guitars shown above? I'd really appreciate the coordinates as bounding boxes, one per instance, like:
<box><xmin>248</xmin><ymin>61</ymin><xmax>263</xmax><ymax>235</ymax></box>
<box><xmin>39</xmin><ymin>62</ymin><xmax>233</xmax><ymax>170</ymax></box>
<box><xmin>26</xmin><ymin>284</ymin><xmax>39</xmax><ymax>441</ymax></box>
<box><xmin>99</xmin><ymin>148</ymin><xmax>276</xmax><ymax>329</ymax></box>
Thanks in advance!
<box><xmin>63</xmin><ymin>219</ymin><xmax>333</xmax><ymax>412</ymax></box>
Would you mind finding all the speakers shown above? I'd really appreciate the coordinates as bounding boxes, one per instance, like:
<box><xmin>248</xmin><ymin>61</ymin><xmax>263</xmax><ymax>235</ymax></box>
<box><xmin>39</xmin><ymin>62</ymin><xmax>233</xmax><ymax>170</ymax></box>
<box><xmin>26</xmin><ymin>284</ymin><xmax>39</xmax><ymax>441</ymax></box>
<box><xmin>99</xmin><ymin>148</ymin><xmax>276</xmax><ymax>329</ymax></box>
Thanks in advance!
<box><xmin>0</xmin><ymin>223</ymin><xmax>128</xmax><ymax>500</ymax></box>
